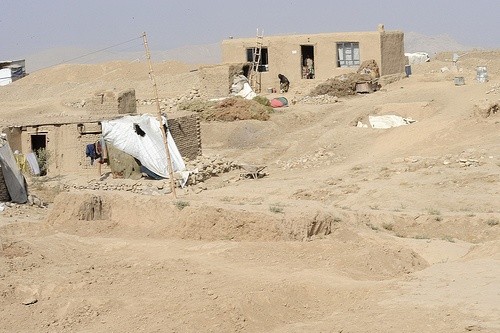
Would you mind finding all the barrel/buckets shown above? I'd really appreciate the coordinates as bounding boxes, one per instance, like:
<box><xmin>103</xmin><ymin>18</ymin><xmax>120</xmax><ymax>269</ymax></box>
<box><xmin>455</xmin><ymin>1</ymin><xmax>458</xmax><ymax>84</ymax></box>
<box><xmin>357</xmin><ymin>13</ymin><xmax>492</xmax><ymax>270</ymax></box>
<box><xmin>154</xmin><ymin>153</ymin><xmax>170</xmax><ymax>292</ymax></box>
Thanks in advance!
<box><xmin>405</xmin><ymin>65</ymin><xmax>411</xmax><ymax>77</ymax></box>
<box><xmin>477</xmin><ymin>65</ymin><xmax>487</xmax><ymax>82</ymax></box>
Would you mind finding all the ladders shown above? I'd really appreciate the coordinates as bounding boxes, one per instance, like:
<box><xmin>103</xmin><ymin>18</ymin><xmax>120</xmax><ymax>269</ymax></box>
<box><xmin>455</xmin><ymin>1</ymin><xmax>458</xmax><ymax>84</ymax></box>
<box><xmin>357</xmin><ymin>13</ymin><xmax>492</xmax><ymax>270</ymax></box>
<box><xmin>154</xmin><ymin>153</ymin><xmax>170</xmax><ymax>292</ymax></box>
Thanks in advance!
<box><xmin>249</xmin><ymin>28</ymin><xmax>264</xmax><ymax>94</ymax></box>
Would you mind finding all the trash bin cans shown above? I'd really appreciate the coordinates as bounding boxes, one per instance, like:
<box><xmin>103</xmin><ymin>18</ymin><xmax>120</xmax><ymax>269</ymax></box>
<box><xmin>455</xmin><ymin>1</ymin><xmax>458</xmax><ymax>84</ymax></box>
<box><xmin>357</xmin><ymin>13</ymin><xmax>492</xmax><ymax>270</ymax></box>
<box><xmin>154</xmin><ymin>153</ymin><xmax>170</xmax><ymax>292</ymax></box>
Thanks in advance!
<box><xmin>477</xmin><ymin>66</ymin><xmax>488</xmax><ymax>83</ymax></box>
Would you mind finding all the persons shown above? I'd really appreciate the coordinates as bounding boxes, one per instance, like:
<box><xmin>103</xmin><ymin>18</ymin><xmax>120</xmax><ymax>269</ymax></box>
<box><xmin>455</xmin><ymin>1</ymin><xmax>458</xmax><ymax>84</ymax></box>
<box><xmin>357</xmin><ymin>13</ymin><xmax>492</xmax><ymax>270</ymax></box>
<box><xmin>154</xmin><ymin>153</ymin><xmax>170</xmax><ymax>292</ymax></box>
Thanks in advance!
<box><xmin>304</xmin><ymin>54</ymin><xmax>314</xmax><ymax>79</ymax></box>
<box><xmin>278</xmin><ymin>74</ymin><xmax>289</xmax><ymax>94</ymax></box>
